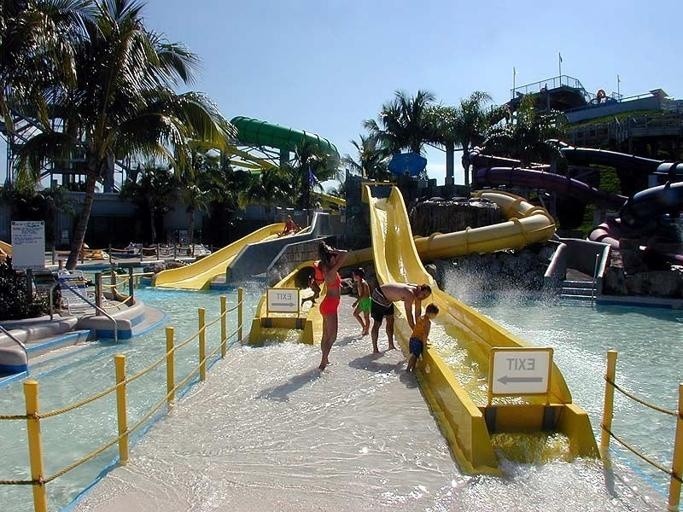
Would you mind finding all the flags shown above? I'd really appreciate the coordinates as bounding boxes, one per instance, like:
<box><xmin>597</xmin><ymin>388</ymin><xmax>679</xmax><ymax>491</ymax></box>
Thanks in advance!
<box><xmin>559</xmin><ymin>53</ymin><xmax>562</xmax><ymax>62</ymax></box>
<box><xmin>309</xmin><ymin>166</ymin><xmax>319</xmax><ymax>188</ymax></box>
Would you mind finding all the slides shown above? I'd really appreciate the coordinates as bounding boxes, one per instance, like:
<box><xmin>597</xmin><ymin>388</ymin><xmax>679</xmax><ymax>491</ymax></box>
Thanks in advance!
<box><xmin>468</xmin><ymin>130</ymin><xmax>683</xmax><ymax>311</ymax></box>
<box><xmin>360</xmin><ymin>179</ymin><xmax>601</xmax><ymax>476</ymax></box>
<box><xmin>246</xmin><ymin>188</ymin><xmax>555</xmax><ymax>346</ymax></box>
<box><xmin>154</xmin><ymin>223</ymin><xmax>285</xmax><ymax>293</ymax></box>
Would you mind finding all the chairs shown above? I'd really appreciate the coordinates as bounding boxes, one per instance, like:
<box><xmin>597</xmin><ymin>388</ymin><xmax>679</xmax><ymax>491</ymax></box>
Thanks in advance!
<box><xmin>57</xmin><ymin>269</ymin><xmax>106</xmax><ymax>314</ymax></box>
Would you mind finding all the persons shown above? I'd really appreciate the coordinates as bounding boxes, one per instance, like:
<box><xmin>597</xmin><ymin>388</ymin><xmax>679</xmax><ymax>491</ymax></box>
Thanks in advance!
<box><xmin>370</xmin><ymin>282</ymin><xmax>431</xmax><ymax>353</ymax></box>
<box><xmin>436</xmin><ymin>176</ymin><xmax>458</xmax><ymax>198</ymax></box>
<box><xmin>399</xmin><ymin>171</ymin><xmax>413</xmax><ymax>185</ymax></box>
<box><xmin>317</xmin><ymin>240</ymin><xmax>349</xmax><ymax>371</ymax></box>
<box><xmin>638</xmin><ymin>239</ymin><xmax>664</xmax><ymax>271</ymax></box>
<box><xmin>276</xmin><ymin>214</ymin><xmax>297</xmax><ymax>237</ymax></box>
<box><xmin>406</xmin><ymin>303</ymin><xmax>439</xmax><ymax>373</ymax></box>
<box><xmin>352</xmin><ymin>267</ymin><xmax>372</xmax><ymax>335</ymax></box>
<box><xmin>128</xmin><ymin>241</ymin><xmax>136</xmax><ymax>250</ymax></box>
